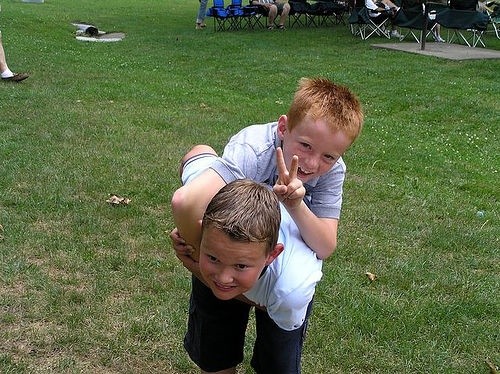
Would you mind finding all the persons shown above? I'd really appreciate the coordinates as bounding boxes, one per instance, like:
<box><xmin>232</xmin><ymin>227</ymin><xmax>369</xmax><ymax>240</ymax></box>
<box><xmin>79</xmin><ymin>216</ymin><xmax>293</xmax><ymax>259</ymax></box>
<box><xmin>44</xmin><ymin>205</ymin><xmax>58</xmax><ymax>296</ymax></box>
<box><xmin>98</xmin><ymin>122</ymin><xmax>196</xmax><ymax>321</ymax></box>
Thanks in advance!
<box><xmin>249</xmin><ymin>0</ymin><xmax>290</xmax><ymax>31</ymax></box>
<box><xmin>0</xmin><ymin>29</ymin><xmax>29</xmax><ymax>82</ymax></box>
<box><xmin>364</xmin><ymin>0</ymin><xmax>445</xmax><ymax>42</ymax></box>
<box><xmin>172</xmin><ymin>77</ymin><xmax>363</xmax><ymax>263</ymax></box>
<box><xmin>195</xmin><ymin>0</ymin><xmax>209</xmax><ymax>30</ymax></box>
<box><xmin>169</xmin><ymin>144</ymin><xmax>323</xmax><ymax>374</ymax></box>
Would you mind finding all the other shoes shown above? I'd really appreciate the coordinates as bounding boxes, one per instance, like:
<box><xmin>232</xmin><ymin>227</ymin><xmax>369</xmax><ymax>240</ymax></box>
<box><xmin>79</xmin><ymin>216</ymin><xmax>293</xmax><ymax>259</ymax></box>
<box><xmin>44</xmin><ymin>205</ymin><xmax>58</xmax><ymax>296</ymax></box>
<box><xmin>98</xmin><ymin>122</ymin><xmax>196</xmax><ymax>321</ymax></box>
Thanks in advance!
<box><xmin>196</xmin><ymin>27</ymin><xmax>203</xmax><ymax>30</ymax></box>
<box><xmin>391</xmin><ymin>30</ymin><xmax>404</xmax><ymax>41</ymax></box>
<box><xmin>1</xmin><ymin>73</ymin><xmax>29</xmax><ymax>82</ymax></box>
<box><xmin>277</xmin><ymin>24</ymin><xmax>286</xmax><ymax>30</ymax></box>
<box><xmin>434</xmin><ymin>36</ymin><xmax>446</xmax><ymax>43</ymax></box>
<box><xmin>268</xmin><ymin>26</ymin><xmax>274</xmax><ymax>30</ymax></box>
<box><xmin>201</xmin><ymin>24</ymin><xmax>206</xmax><ymax>27</ymax></box>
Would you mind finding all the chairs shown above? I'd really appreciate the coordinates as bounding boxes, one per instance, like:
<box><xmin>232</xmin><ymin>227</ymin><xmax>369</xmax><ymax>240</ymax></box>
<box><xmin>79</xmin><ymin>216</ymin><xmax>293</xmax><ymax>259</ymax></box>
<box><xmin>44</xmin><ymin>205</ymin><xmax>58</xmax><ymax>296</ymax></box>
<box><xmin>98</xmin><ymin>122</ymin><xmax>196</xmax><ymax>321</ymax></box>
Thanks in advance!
<box><xmin>205</xmin><ymin>0</ymin><xmax>500</xmax><ymax>50</ymax></box>
